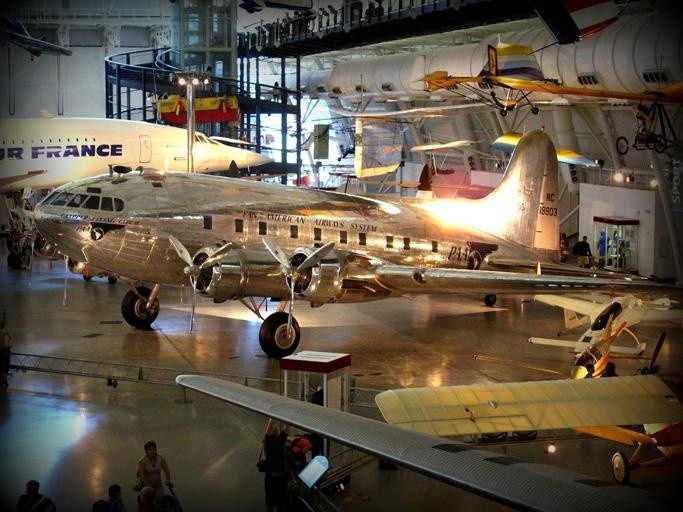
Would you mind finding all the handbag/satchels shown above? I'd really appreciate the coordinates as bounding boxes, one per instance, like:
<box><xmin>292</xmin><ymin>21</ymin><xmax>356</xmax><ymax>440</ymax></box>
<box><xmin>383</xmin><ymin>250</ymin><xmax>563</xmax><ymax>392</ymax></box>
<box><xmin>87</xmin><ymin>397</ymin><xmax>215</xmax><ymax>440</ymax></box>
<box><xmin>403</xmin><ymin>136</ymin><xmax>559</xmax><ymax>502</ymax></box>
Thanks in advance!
<box><xmin>256</xmin><ymin>461</ymin><xmax>266</xmax><ymax>473</ymax></box>
<box><xmin>130</xmin><ymin>479</ymin><xmax>141</xmax><ymax>491</ymax></box>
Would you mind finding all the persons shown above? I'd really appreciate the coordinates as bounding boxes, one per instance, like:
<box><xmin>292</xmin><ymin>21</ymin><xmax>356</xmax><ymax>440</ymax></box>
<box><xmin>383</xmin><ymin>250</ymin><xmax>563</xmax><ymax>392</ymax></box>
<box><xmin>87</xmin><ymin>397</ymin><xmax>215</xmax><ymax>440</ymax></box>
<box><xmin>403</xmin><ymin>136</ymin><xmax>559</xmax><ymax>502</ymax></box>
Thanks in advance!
<box><xmin>560</xmin><ymin>232</ymin><xmax>570</xmax><ymax>263</ymax></box>
<box><xmin>16</xmin><ymin>415</ymin><xmax>315</xmax><ymax>512</ymax></box>
<box><xmin>272</xmin><ymin>82</ymin><xmax>281</xmax><ymax>103</ymax></box>
<box><xmin>0</xmin><ymin>309</ymin><xmax>11</xmax><ymax>386</ymax></box>
<box><xmin>280</xmin><ymin>82</ymin><xmax>287</xmax><ymax>104</ymax></box>
<box><xmin>574</xmin><ymin>235</ymin><xmax>591</xmax><ymax>267</ymax></box>
<box><xmin>612</xmin><ymin>230</ymin><xmax>625</xmax><ymax>269</ymax></box>
<box><xmin>597</xmin><ymin>230</ymin><xmax>609</xmax><ymax>266</ymax></box>
<box><xmin>206</xmin><ymin>66</ymin><xmax>214</xmax><ymax>96</ymax></box>
<box><xmin>267</xmin><ymin>10</ymin><xmax>305</xmax><ymax>43</ymax></box>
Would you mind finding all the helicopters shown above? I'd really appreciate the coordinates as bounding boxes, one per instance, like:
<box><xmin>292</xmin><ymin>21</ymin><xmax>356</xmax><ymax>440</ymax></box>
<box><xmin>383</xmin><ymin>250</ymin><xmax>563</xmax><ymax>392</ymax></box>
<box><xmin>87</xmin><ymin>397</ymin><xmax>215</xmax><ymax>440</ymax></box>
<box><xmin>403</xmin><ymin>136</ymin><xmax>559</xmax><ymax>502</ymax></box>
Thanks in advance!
<box><xmin>146</xmin><ymin>64</ymin><xmax>304</xmax><ymax>128</ymax></box>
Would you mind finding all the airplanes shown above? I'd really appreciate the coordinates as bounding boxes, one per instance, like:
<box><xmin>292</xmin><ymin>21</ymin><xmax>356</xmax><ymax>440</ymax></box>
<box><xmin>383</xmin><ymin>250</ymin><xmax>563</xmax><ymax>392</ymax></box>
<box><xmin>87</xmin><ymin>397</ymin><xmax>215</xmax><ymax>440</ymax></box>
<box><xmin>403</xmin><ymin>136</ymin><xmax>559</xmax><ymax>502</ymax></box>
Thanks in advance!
<box><xmin>528</xmin><ymin>334</ymin><xmax>670</xmax><ymax>383</ymax></box>
<box><xmin>413</xmin><ymin>12</ymin><xmax>683</xmax><ymax>117</ymax></box>
<box><xmin>533</xmin><ymin>292</ymin><xmax>683</xmax><ymax>355</ymax></box>
<box><xmin>0</xmin><ymin>114</ymin><xmax>279</xmax><ymax>270</ymax></box>
<box><xmin>5</xmin><ymin>128</ymin><xmax>673</xmax><ymax>359</ymax></box>
<box><xmin>170</xmin><ymin>371</ymin><xmax>681</xmax><ymax>510</ymax></box>
<box><xmin>490</xmin><ymin>132</ymin><xmax>601</xmax><ymax>169</ymax></box>
<box><xmin>372</xmin><ymin>366</ymin><xmax>683</xmax><ymax>487</ymax></box>
